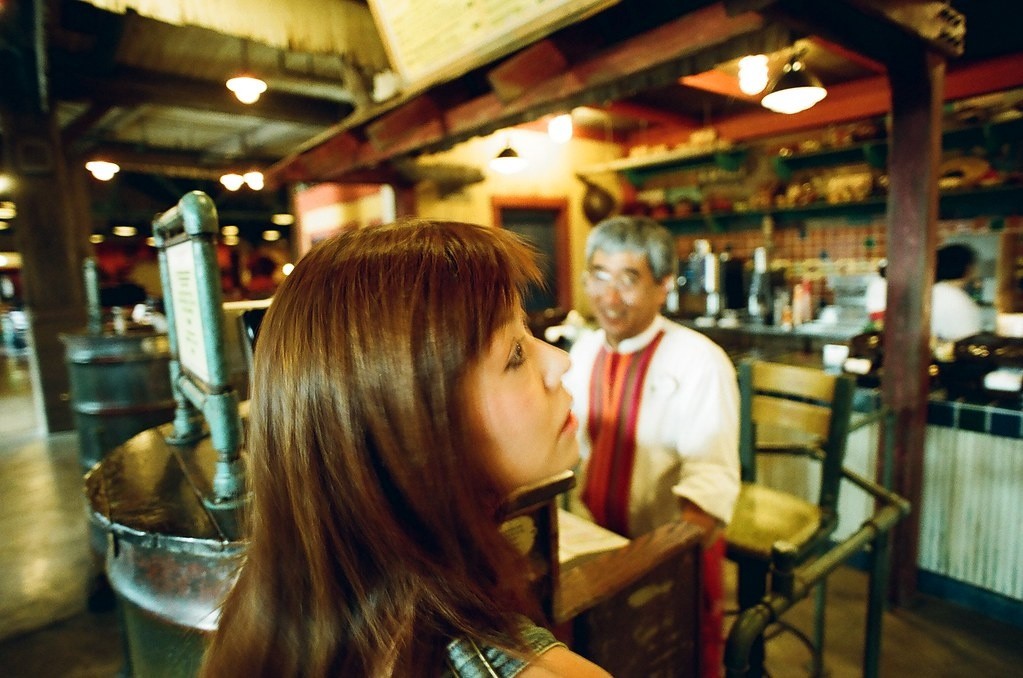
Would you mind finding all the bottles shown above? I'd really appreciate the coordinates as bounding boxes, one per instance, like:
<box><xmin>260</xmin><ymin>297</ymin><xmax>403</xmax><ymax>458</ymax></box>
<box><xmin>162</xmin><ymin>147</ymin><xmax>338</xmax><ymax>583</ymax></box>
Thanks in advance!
<box><xmin>661</xmin><ymin>241</ymin><xmax>827</xmax><ymax>333</ymax></box>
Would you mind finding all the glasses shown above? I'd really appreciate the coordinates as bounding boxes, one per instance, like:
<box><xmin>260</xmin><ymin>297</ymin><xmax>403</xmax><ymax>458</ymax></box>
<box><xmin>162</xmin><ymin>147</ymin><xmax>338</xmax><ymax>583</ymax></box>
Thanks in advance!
<box><xmin>585</xmin><ymin>270</ymin><xmax>654</xmax><ymax>294</ymax></box>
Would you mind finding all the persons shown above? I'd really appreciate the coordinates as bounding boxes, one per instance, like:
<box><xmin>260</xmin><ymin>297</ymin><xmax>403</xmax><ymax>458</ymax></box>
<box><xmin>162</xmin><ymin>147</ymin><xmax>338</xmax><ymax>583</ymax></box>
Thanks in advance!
<box><xmin>219</xmin><ymin>256</ymin><xmax>283</xmax><ymax>338</ymax></box>
<box><xmin>561</xmin><ymin>215</ymin><xmax>740</xmax><ymax>678</ymax></box>
<box><xmin>195</xmin><ymin>218</ymin><xmax>625</xmax><ymax>678</ymax></box>
<box><xmin>929</xmin><ymin>243</ymin><xmax>982</xmax><ymax>362</ymax></box>
<box><xmin>107</xmin><ymin>268</ymin><xmax>147</xmax><ymax>311</ymax></box>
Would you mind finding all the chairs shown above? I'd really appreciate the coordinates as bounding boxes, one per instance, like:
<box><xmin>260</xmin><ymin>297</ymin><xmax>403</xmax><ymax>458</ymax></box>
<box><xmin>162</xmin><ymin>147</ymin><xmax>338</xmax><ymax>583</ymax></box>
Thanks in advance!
<box><xmin>720</xmin><ymin>358</ymin><xmax>854</xmax><ymax>678</ymax></box>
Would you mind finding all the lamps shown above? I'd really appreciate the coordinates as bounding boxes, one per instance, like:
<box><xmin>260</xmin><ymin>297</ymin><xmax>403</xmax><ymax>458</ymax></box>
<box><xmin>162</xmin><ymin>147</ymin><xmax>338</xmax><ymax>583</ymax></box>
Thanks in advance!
<box><xmin>85</xmin><ymin>149</ymin><xmax>121</xmax><ymax>181</ymax></box>
<box><xmin>243</xmin><ymin>163</ymin><xmax>265</xmax><ymax>190</ymax></box>
<box><xmin>220</xmin><ymin>166</ymin><xmax>244</xmax><ymax>192</ymax></box>
<box><xmin>760</xmin><ymin>52</ymin><xmax>827</xmax><ymax>115</ymax></box>
<box><xmin>489</xmin><ymin>133</ymin><xmax>527</xmax><ymax>174</ymax></box>
<box><xmin>225</xmin><ymin>66</ymin><xmax>268</xmax><ymax>104</ymax></box>
